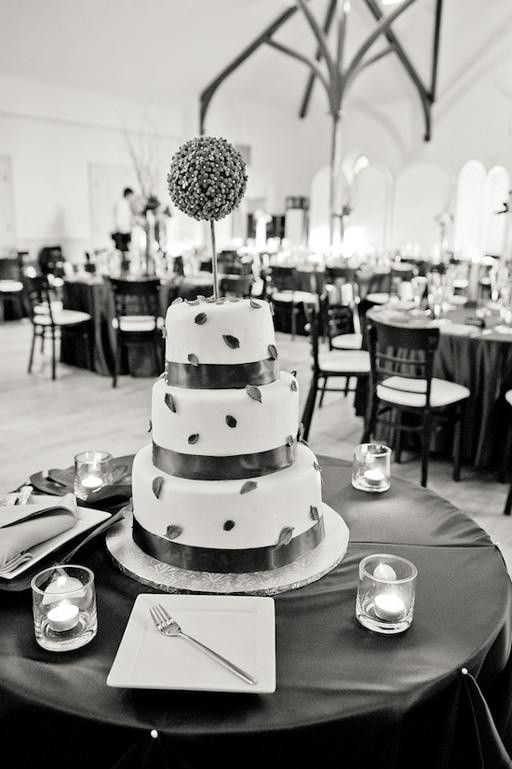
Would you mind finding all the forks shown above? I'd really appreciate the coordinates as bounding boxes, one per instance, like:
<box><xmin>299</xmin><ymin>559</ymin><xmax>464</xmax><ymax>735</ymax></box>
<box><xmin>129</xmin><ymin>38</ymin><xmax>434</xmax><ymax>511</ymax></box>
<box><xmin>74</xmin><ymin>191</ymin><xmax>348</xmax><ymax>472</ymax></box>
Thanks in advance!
<box><xmin>149</xmin><ymin>604</ymin><xmax>256</xmax><ymax>686</ymax></box>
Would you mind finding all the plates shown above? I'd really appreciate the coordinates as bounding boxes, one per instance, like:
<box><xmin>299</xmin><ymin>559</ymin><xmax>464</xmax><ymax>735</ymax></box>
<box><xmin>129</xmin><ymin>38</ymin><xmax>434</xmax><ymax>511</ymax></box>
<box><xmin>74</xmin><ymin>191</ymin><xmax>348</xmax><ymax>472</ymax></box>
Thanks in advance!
<box><xmin>0</xmin><ymin>492</ymin><xmax>112</xmax><ymax>579</ymax></box>
<box><xmin>105</xmin><ymin>593</ymin><xmax>277</xmax><ymax>695</ymax></box>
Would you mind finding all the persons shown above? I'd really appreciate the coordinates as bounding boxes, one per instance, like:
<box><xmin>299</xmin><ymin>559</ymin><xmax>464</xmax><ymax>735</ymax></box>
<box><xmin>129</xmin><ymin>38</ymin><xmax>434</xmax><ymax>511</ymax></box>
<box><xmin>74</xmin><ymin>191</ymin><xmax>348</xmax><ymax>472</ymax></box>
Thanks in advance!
<box><xmin>110</xmin><ymin>186</ymin><xmax>137</xmax><ymax>276</ymax></box>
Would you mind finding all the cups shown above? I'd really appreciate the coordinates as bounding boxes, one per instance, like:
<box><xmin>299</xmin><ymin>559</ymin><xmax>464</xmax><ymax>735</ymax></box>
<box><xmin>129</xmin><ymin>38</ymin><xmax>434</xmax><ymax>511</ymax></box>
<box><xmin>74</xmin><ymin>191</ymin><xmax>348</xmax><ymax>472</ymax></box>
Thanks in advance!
<box><xmin>351</xmin><ymin>443</ymin><xmax>391</xmax><ymax>493</ymax></box>
<box><xmin>357</xmin><ymin>553</ymin><xmax>418</xmax><ymax>635</ymax></box>
<box><xmin>74</xmin><ymin>449</ymin><xmax>113</xmax><ymax>502</ymax></box>
<box><xmin>31</xmin><ymin>565</ymin><xmax>99</xmax><ymax>654</ymax></box>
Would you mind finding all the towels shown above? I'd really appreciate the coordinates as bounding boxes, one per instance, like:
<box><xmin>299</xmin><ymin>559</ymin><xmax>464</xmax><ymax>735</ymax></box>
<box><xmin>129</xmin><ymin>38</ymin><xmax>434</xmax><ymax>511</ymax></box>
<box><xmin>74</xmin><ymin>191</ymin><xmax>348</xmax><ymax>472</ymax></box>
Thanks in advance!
<box><xmin>0</xmin><ymin>492</ymin><xmax>79</xmax><ymax>575</ymax></box>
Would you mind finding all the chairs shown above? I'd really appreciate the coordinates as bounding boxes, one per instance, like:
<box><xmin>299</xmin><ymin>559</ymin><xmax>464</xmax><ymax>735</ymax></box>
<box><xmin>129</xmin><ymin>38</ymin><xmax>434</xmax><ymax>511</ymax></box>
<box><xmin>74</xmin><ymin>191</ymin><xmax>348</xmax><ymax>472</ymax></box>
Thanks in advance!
<box><xmin>0</xmin><ymin>252</ymin><xmax>31</xmax><ymax>324</ymax></box>
<box><xmin>367</xmin><ymin>266</ymin><xmax>416</xmax><ymax>306</ymax></box>
<box><xmin>101</xmin><ymin>274</ymin><xmax>164</xmax><ymax>388</ymax></box>
<box><xmin>17</xmin><ymin>273</ymin><xmax>91</xmax><ymax>379</ymax></box>
<box><xmin>360</xmin><ymin>311</ymin><xmax>470</xmax><ymax>487</ymax></box>
<box><xmin>318</xmin><ymin>293</ymin><xmax>363</xmax><ymax>408</ymax></box>
<box><xmin>298</xmin><ymin>305</ymin><xmax>372</xmax><ymax>441</ymax></box>
<box><xmin>268</xmin><ymin>266</ymin><xmax>314</xmax><ymax>343</ymax></box>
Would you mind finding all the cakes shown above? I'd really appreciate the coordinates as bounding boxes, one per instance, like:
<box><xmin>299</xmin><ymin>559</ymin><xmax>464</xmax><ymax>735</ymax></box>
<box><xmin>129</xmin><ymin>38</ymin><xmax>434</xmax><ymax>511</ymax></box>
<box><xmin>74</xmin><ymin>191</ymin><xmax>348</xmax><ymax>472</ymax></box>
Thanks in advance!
<box><xmin>131</xmin><ymin>298</ymin><xmax>327</xmax><ymax>576</ymax></box>
